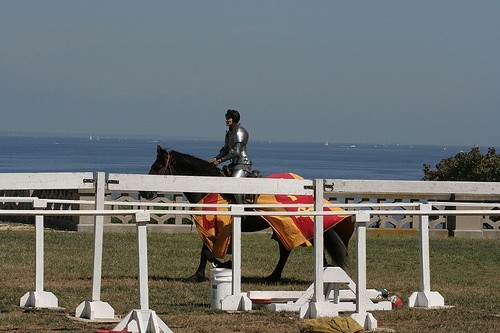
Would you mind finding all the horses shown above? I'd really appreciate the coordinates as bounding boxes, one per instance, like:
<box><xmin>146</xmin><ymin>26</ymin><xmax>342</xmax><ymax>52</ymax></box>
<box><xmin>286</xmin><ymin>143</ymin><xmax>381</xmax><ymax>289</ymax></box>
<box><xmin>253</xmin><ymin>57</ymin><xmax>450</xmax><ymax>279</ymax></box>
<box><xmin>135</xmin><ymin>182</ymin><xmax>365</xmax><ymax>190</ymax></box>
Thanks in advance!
<box><xmin>139</xmin><ymin>146</ymin><xmax>351</xmax><ymax>284</ymax></box>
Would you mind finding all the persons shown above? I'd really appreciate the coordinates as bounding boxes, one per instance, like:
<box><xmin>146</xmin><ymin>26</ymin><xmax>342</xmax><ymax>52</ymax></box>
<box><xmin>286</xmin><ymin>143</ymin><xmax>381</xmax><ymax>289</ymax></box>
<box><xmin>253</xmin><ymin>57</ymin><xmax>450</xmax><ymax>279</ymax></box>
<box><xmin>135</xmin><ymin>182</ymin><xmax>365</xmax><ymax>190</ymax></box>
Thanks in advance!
<box><xmin>208</xmin><ymin>109</ymin><xmax>252</xmax><ymax>205</ymax></box>
